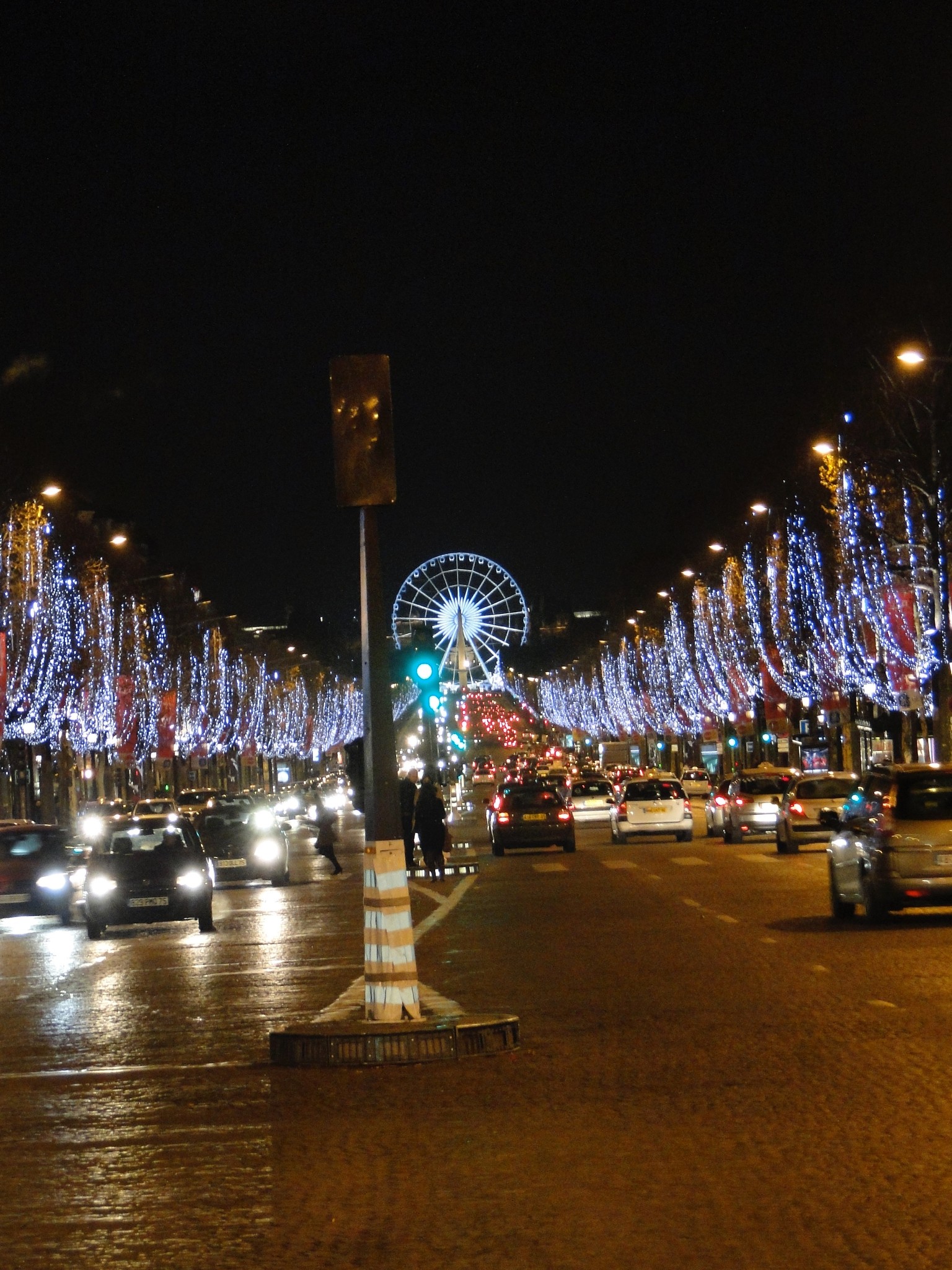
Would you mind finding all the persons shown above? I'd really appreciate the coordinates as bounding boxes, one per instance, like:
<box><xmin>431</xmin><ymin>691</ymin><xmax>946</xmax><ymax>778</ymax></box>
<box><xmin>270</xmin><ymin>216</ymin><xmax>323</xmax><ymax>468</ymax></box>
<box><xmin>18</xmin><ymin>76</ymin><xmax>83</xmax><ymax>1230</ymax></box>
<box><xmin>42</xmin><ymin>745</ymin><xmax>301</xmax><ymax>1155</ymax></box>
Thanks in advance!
<box><xmin>300</xmin><ymin>763</ymin><xmax>467</xmax><ymax>884</ymax></box>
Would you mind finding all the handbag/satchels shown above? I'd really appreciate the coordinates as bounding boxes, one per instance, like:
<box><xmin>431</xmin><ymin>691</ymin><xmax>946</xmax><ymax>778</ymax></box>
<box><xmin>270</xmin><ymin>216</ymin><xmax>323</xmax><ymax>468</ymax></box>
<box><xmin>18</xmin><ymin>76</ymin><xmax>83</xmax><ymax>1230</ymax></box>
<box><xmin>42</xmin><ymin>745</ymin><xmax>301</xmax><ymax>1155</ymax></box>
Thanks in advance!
<box><xmin>435</xmin><ymin>817</ymin><xmax>446</xmax><ymax>846</ymax></box>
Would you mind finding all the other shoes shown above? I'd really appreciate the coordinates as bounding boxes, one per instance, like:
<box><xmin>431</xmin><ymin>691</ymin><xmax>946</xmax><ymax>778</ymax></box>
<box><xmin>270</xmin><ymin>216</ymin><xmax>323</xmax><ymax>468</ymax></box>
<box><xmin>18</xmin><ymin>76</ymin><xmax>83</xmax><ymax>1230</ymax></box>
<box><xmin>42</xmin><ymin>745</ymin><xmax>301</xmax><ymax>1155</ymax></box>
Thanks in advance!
<box><xmin>408</xmin><ymin>861</ymin><xmax>419</xmax><ymax>867</ymax></box>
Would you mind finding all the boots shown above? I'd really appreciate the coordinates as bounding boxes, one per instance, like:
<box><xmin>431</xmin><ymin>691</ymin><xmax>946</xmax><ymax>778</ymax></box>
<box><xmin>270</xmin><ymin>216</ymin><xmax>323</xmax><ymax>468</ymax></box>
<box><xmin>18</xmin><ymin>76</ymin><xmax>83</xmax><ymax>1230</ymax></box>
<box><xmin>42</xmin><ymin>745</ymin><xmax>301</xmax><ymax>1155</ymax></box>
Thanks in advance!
<box><xmin>430</xmin><ymin>866</ymin><xmax>438</xmax><ymax>883</ymax></box>
<box><xmin>439</xmin><ymin>865</ymin><xmax>446</xmax><ymax>882</ymax></box>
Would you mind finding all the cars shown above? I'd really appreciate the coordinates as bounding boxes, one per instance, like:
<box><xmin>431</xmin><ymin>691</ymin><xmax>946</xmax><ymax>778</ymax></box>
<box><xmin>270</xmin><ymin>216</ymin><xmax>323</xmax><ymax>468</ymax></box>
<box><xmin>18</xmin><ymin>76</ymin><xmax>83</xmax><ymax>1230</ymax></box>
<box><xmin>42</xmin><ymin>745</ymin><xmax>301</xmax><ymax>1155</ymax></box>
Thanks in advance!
<box><xmin>490</xmin><ymin>783</ymin><xmax>578</xmax><ymax>855</ymax></box>
<box><xmin>0</xmin><ymin>770</ymin><xmax>355</xmax><ymax>919</ymax></box>
<box><xmin>723</xmin><ymin>762</ymin><xmax>800</xmax><ymax>843</ymax></box>
<box><xmin>772</xmin><ymin>771</ymin><xmax>864</xmax><ymax>853</ymax></box>
<box><xmin>77</xmin><ymin>814</ymin><xmax>214</xmax><ymax>934</ymax></box>
<box><xmin>703</xmin><ymin>778</ymin><xmax>731</xmax><ymax>837</ymax></box>
<box><xmin>462</xmin><ymin>743</ymin><xmax>713</xmax><ymax>802</ymax></box>
<box><xmin>605</xmin><ymin>775</ymin><xmax>694</xmax><ymax>844</ymax></box>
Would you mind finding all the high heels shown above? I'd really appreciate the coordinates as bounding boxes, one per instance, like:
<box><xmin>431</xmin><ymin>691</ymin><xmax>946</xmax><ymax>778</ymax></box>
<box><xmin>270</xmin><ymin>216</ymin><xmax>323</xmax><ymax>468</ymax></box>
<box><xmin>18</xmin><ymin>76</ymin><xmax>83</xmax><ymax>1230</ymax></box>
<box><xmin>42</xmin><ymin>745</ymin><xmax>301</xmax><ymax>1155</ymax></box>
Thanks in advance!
<box><xmin>330</xmin><ymin>864</ymin><xmax>343</xmax><ymax>875</ymax></box>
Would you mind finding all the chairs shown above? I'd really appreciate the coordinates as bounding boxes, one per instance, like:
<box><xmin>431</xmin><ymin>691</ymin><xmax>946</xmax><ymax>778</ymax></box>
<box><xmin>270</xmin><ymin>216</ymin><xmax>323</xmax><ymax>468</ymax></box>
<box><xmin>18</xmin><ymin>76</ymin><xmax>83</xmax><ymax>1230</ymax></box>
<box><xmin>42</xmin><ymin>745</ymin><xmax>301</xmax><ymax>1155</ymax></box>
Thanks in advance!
<box><xmin>207</xmin><ymin>817</ymin><xmax>223</xmax><ymax>829</ymax></box>
<box><xmin>113</xmin><ymin>837</ymin><xmax>132</xmax><ymax>852</ymax></box>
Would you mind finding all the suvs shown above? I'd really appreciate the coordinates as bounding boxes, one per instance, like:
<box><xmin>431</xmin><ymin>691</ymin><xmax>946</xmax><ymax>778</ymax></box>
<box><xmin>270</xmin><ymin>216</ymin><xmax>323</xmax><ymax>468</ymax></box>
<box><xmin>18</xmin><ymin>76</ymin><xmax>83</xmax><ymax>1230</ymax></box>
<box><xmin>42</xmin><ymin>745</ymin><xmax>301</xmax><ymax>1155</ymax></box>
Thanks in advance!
<box><xmin>818</xmin><ymin>758</ymin><xmax>952</xmax><ymax>921</ymax></box>
